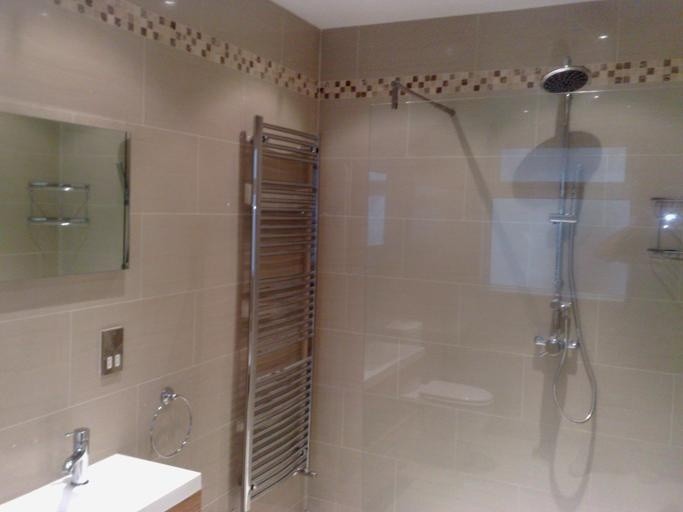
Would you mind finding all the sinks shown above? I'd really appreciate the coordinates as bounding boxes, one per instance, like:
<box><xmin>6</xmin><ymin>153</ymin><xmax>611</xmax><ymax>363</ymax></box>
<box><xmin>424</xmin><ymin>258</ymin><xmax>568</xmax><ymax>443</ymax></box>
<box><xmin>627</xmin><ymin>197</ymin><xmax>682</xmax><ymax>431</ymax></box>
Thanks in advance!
<box><xmin>0</xmin><ymin>454</ymin><xmax>202</xmax><ymax>511</ymax></box>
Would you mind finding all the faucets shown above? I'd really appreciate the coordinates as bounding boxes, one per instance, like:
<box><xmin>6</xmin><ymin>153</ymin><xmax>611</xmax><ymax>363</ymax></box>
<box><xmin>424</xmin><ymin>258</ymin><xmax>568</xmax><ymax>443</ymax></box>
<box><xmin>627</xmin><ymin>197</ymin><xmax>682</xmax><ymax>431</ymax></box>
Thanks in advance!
<box><xmin>61</xmin><ymin>427</ymin><xmax>91</xmax><ymax>485</ymax></box>
